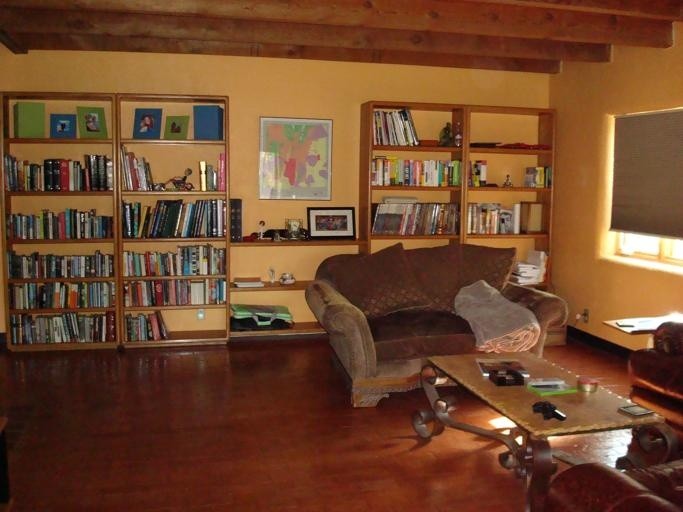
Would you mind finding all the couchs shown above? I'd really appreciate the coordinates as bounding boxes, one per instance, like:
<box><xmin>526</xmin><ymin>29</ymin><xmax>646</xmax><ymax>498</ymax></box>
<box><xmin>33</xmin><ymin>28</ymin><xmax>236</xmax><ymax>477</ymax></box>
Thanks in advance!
<box><xmin>544</xmin><ymin>456</ymin><xmax>683</xmax><ymax>512</ymax></box>
<box><xmin>305</xmin><ymin>249</ymin><xmax>568</xmax><ymax>408</ymax></box>
<box><xmin>627</xmin><ymin>323</ymin><xmax>683</xmax><ymax>429</ymax></box>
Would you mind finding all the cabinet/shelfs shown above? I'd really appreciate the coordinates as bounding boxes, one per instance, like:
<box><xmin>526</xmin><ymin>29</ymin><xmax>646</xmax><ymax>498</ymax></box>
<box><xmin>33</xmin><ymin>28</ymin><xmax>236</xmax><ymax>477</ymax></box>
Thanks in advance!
<box><xmin>0</xmin><ymin>90</ymin><xmax>120</xmax><ymax>353</ymax></box>
<box><xmin>464</xmin><ymin>104</ymin><xmax>557</xmax><ymax>294</ymax></box>
<box><xmin>359</xmin><ymin>100</ymin><xmax>467</xmax><ymax>255</ymax></box>
<box><xmin>116</xmin><ymin>94</ymin><xmax>231</xmax><ymax>348</ymax></box>
<box><xmin>232</xmin><ymin>234</ymin><xmax>360</xmax><ymax>343</ymax></box>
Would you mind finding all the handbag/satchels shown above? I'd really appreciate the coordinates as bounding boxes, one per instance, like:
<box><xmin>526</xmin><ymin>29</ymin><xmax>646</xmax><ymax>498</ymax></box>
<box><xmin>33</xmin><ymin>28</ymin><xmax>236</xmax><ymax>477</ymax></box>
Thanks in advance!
<box><xmin>231</xmin><ymin>303</ymin><xmax>293</xmax><ymax>326</ymax></box>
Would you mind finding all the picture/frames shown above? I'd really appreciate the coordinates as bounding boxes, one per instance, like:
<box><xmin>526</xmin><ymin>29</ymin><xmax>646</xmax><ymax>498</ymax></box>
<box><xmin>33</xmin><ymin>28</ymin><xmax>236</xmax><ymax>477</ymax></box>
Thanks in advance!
<box><xmin>307</xmin><ymin>207</ymin><xmax>357</xmax><ymax>240</ymax></box>
<box><xmin>258</xmin><ymin>116</ymin><xmax>333</xmax><ymax>201</ymax></box>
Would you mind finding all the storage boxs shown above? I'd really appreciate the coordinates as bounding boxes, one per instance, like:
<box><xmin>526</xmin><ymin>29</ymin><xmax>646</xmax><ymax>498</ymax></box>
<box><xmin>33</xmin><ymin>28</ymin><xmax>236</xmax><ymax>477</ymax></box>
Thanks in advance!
<box><xmin>191</xmin><ymin>104</ymin><xmax>224</xmax><ymax>139</ymax></box>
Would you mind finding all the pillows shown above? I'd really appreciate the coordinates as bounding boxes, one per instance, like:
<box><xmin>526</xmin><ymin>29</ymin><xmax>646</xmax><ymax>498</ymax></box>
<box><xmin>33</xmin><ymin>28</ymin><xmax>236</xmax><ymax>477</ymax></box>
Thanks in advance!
<box><xmin>462</xmin><ymin>243</ymin><xmax>517</xmax><ymax>296</ymax></box>
<box><xmin>404</xmin><ymin>245</ymin><xmax>461</xmax><ymax>315</ymax></box>
<box><xmin>329</xmin><ymin>243</ymin><xmax>432</xmax><ymax>321</ymax></box>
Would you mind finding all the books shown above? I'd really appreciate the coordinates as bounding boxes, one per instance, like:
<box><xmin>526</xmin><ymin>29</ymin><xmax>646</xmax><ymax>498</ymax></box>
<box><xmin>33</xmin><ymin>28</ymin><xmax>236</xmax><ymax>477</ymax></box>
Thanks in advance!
<box><xmin>10</xmin><ymin>311</ymin><xmax>115</xmax><ymax>345</ymax></box>
<box><xmin>122</xmin><ymin>199</ymin><xmax>226</xmax><ymax>239</ymax></box>
<box><xmin>509</xmin><ymin>250</ymin><xmax>548</xmax><ymax>285</ymax></box>
<box><xmin>199</xmin><ymin>153</ymin><xmax>225</xmax><ymax>192</ymax></box>
<box><xmin>9</xmin><ymin>281</ymin><xmax>115</xmax><ymax>309</ymax></box>
<box><xmin>371</xmin><ymin>203</ymin><xmax>458</xmax><ymax>236</ymax></box>
<box><xmin>122</xmin><ymin>243</ymin><xmax>225</xmax><ymax>276</ymax></box>
<box><xmin>6</xmin><ymin>208</ymin><xmax>112</xmax><ymax>239</ymax></box>
<box><xmin>469</xmin><ymin>159</ymin><xmax>487</xmax><ymax>187</ymax></box>
<box><xmin>124</xmin><ymin>279</ymin><xmax>226</xmax><ymax>307</ymax></box>
<box><xmin>7</xmin><ymin>250</ymin><xmax>114</xmax><ymax>279</ymax></box>
<box><xmin>120</xmin><ymin>146</ymin><xmax>154</xmax><ymax>192</ymax></box>
<box><xmin>524</xmin><ymin>167</ymin><xmax>550</xmax><ymax>188</ymax></box>
<box><xmin>467</xmin><ymin>202</ymin><xmax>500</xmax><ymax>235</ymax></box>
<box><xmin>4</xmin><ymin>154</ymin><xmax>113</xmax><ymax>192</ymax></box>
<box><xmin>372</xmin><ymin>109</ymin><xmax>419</xmax><ymax>146</ymax></box>
<box><xmin>371</xmin><ymin>155</ymin><xmax>460</xmax><ymax>187</ymax></box>
<box><xmin>125</xmin><ymin>310</ymin><xmax>168</xmax><ymax>341</ymax></box>
